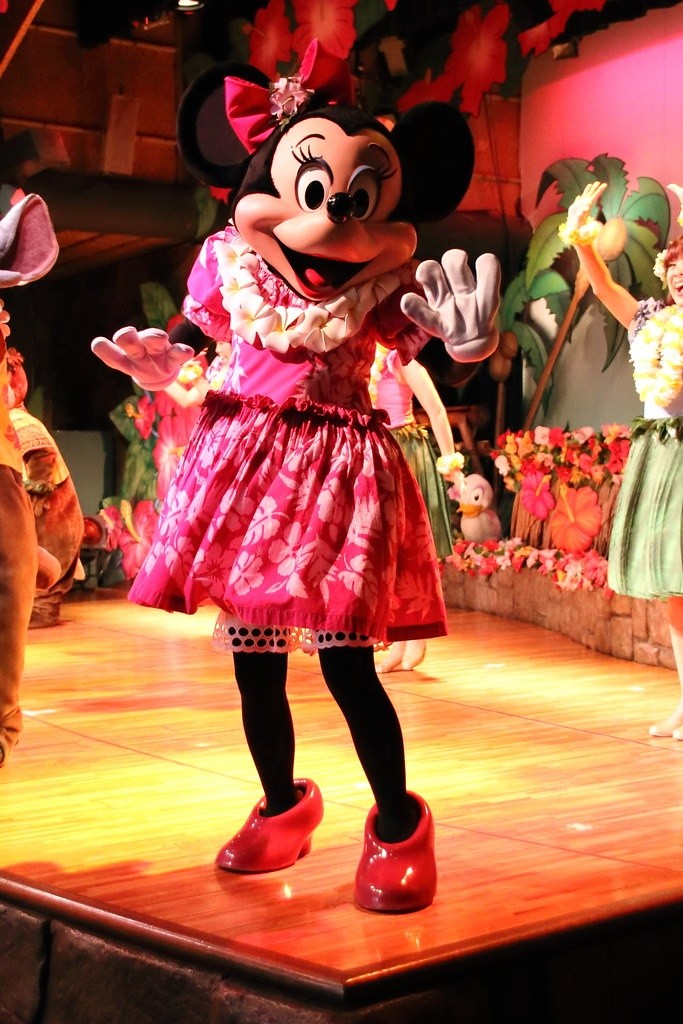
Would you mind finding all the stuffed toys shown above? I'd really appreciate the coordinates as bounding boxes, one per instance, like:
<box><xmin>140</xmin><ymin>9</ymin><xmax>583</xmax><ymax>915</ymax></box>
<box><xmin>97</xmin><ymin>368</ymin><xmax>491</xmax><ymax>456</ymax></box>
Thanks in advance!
<box><xmin>89</xmin><ymin>45</ymin><xmax>505</xmax><ymax>915</ymax></box>
<box><xmin>0</xmin><ymin>189</ymin><xmax>84</xmax><ymax>765</ymax></box>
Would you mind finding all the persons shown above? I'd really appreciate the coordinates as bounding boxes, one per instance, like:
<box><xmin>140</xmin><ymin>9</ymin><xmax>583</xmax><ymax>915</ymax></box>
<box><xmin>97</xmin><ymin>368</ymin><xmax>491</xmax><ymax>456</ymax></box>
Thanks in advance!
<box><xmin>354</xmin><ymin>334</ymin><xmax>469</xmax><ymax>676</ymax></box>
<box><xmin>559</xmin><ymin>180</ymin><xmax>683</xmax><ymax>740</ymax></box>
